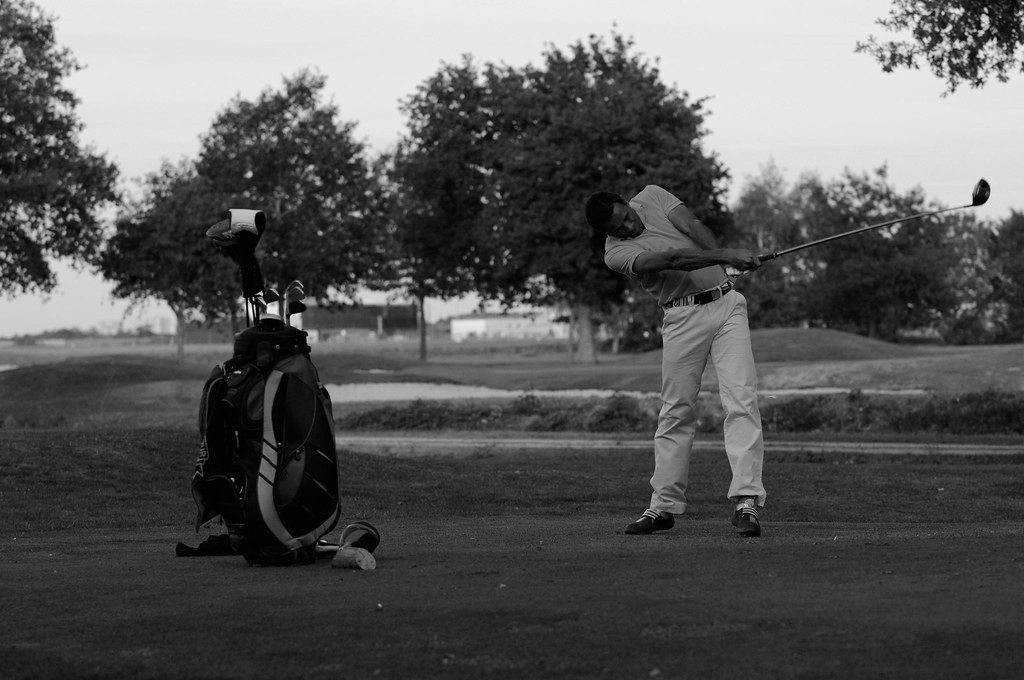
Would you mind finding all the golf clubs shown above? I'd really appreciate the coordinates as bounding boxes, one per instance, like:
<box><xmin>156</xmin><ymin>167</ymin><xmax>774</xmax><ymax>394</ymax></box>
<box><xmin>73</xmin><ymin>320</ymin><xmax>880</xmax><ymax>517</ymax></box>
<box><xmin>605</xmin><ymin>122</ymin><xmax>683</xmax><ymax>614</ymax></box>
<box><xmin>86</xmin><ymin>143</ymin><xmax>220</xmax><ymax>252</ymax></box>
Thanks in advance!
<box><xmin>759</xmin><ymin>179</ymin><xmax>990</xmax><ymax>263</ymax></box>
<box><xmin>249</xmin><ymin>282</ymin><xmax>307</xmax><ymax>319</ymax></box>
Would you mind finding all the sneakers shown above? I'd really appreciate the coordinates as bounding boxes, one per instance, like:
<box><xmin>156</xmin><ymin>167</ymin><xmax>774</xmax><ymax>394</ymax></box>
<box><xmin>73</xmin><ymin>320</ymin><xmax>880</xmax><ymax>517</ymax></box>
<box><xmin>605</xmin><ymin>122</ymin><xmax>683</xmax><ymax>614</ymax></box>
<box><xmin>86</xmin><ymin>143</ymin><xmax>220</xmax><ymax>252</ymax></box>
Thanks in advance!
<box><xmin>625</xmin><ymin>509</ymin><xmax>674</xmax><ymax>535</ymax></box>
<box><xmin>730</xmin><ymin>503</ymin><xmax>762</xmax><ymax>536</ymax></box>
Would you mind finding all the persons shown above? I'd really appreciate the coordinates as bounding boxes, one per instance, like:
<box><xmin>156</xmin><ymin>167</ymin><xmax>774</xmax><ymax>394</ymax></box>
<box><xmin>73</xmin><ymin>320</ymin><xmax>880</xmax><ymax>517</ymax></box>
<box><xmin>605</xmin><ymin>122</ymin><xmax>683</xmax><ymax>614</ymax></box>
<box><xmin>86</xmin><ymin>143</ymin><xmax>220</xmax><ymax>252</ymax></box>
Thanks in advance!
<box><xmin>586</xmin><ymin>185</ymin><xmax>767</xmax><ymax>536</ymax></box>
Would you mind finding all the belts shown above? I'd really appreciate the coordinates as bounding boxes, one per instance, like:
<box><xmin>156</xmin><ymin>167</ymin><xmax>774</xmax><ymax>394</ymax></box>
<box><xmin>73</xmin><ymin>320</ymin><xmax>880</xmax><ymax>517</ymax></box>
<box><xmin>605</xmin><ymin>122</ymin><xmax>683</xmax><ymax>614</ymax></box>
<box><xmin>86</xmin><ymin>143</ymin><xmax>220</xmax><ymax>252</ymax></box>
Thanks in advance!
<box><xmin>661</xmin><ymin>280</ymin><xmax>731</xmax><ymax>309</ymax></box>
